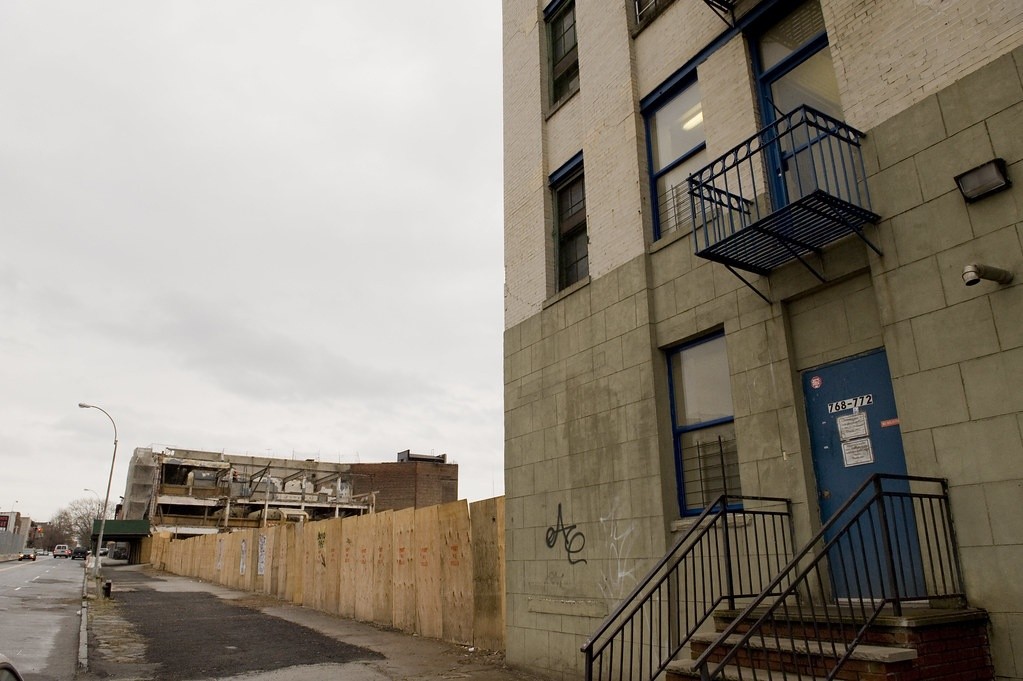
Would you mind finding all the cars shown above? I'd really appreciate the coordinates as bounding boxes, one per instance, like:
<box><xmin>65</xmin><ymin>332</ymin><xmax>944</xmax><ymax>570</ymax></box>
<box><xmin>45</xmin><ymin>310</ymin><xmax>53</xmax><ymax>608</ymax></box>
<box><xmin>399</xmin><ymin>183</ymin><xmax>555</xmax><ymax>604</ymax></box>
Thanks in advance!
<box><xmin>36</xmin><ymin>549</ymin><xmax>53</xmax><ymax>557</ymax></box>
<box><xmin>19</xmin><ymin>548</ymin><xmax>35</xmax><ymax>561</ymax></box>
<box><xmin>72</xmin><ymin>547</ymin><xmax>87</xmax><ymax>559</ymax></box>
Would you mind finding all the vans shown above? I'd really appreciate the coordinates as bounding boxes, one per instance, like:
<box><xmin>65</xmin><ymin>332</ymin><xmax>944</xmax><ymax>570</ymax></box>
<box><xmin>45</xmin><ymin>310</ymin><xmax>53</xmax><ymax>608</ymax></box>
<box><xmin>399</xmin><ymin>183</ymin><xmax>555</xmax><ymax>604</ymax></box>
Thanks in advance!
<box><xmin>88</xmin><ymin>548</ymin><xmax>108</xmax><ymax>554</ymax></box>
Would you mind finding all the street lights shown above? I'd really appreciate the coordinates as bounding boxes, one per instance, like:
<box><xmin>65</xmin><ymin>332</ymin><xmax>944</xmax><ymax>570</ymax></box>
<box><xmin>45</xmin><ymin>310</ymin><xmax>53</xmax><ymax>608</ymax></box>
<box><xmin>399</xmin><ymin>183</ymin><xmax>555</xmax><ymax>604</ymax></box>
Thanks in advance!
<box><xmin>83</xmin><ymin>489</ymin><xmax>102</xmax><ymax>518</ymax></box>
<box><xmin>79</xmin><ymin>403</ymin><xmax>118</xmax><ymax>577</ymax></box>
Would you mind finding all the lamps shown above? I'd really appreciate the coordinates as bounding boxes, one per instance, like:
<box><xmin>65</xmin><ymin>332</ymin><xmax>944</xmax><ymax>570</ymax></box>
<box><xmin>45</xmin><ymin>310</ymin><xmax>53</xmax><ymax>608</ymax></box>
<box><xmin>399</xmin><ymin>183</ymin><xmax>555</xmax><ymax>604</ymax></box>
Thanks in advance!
<box><xmin>681</xmin><ymin>112</ymin><xmax>703</xmax><ymax>131</ymax></box>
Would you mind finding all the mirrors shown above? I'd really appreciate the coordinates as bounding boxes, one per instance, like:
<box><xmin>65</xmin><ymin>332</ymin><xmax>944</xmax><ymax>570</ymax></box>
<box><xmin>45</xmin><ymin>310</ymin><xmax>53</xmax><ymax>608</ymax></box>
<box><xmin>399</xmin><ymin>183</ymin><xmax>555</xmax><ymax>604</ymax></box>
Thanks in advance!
<box><xmin>953</xmin><ymin>158</ymin><xmax>1012</xmax><ymax>204</ymax></box>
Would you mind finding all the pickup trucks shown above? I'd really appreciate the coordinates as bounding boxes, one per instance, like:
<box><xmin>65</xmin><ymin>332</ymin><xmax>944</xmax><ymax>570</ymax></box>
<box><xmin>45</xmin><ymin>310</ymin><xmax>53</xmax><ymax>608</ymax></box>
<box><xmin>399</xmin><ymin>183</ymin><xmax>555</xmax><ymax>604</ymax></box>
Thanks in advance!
<box><xmin>53</xmin><ymin>544</ymin><xmax>71</xmax><ymax>558</ymax></box>
